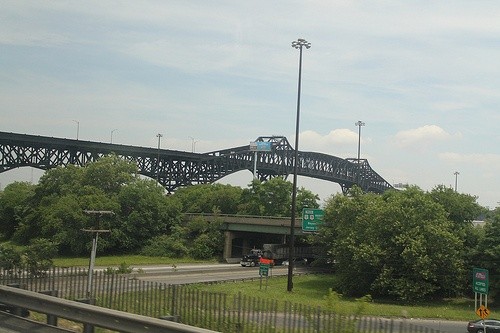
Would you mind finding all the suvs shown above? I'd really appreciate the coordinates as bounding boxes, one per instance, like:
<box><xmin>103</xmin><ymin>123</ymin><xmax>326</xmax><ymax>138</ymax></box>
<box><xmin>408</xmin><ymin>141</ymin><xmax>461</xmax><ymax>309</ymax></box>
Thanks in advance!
<box><xmin>241</xmin><ymin>248</ymin><xmax>274</xmax><ymax>268</ymax></box>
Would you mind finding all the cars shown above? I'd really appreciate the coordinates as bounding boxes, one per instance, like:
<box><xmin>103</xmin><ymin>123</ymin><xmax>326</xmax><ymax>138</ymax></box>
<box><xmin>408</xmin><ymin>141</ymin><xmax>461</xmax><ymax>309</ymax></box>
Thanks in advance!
<box><xmin>467</xmin><ymin>319</ymin><xmax>500</xmax><ymax>333</ymax></box>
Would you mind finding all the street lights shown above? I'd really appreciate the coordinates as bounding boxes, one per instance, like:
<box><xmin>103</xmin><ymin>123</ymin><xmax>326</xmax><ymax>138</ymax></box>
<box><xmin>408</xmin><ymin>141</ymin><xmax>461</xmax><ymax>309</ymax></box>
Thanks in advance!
<box><xmin>287</xmin><ymin>37</ymin><xmax>312</xmax><ymax>291</ymax></box>
<box><xmin>355</xmin><ymin>120</ymin><xmax>366</xmax><ymax>188</ymax></box>
<box><xmin>453</xmin><ymin>171</ymin><xmax>460</xmax><ymax>191</ymax></box>
